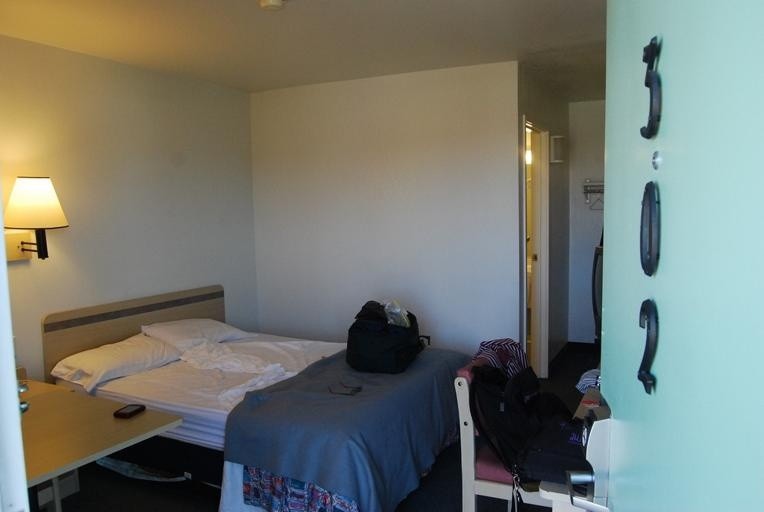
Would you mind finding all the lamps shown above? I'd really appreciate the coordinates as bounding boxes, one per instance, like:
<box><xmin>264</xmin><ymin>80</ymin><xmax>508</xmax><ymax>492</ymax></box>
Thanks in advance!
<box><xmin>2</xmin><ymin>176</ymin><xmax>69</xmax><ymax>261</ymax></box>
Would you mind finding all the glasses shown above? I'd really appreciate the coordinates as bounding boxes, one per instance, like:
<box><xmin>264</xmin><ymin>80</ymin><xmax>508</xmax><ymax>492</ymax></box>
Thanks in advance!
<box><xmin>327</xmin><ymin>382</ymin><xmax>362</xmax><ymax>396</ymax></box>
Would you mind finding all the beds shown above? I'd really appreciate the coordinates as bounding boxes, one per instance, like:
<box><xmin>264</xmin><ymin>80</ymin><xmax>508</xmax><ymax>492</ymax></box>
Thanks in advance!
<box><xmin>41</xmin><ymin>284</ymin><xmax>465</xmax><ymax>511</ymax></box>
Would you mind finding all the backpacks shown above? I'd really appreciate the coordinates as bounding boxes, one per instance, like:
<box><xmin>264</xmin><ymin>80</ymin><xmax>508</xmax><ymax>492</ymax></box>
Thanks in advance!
<box><xmin>468</xmin><ymin>363</ymin><xmax>585</xmax><ymax>484</ymax></box>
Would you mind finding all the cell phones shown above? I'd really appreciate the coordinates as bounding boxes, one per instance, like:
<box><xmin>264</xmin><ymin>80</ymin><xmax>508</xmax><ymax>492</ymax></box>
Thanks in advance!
<box><xmin>114</xmin><ymin>404</ymin><xmax>145</xmax><ymax>418</ymax></box>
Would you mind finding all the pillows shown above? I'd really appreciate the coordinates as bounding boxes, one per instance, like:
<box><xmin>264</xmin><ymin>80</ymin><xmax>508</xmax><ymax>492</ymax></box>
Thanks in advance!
<box><xmin>49</xmin><ymin>317</ymin><xmax>258</xmax><ymax>394</ymax></box>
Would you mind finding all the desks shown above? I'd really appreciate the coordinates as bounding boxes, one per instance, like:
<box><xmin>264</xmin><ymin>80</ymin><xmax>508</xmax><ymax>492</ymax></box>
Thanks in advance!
<box><xmin>17</xmin><ymin>377</ymin><xmax>184</xmax><ymax>511</ymax></box>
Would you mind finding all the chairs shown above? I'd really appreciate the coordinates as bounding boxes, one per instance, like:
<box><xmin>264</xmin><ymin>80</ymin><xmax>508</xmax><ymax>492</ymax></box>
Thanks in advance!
<box><xmin>454</xmin><ymin>339</ymin><xmax>594</xmax><ymax>512</ymax></box>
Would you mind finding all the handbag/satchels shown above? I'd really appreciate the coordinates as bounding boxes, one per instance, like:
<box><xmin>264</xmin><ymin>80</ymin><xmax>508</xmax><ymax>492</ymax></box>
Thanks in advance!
<box><xmin>347</xmin><ymin>300</ymin><xmax>430</xmax><ymax>374</ymax></box>
